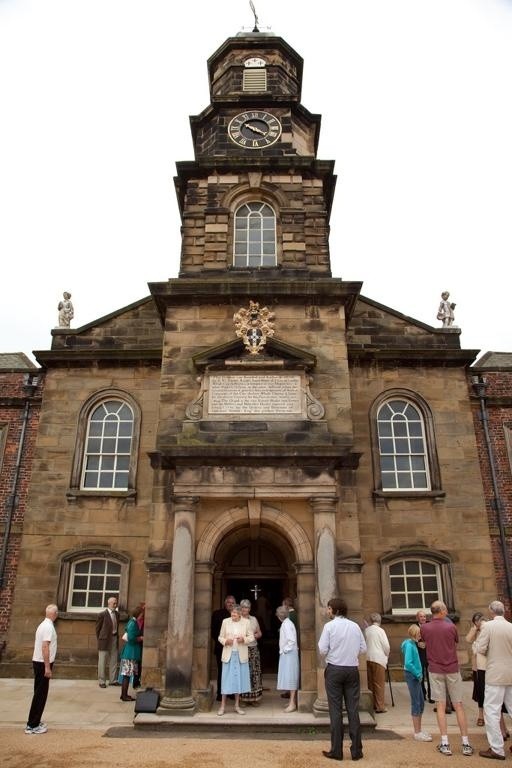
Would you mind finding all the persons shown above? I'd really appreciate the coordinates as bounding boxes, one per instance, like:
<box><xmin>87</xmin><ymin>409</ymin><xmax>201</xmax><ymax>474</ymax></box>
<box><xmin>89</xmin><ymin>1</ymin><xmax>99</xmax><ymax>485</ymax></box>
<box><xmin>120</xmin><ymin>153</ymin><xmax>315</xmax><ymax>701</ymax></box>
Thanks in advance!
<box><xmin>436</xmin><ymin>290</ymin><xmax>457</xmax><ymax>328</ymax></box>
<box><xmin>400</xmin><ymin>597</ymin><xmax>512</xmax><ymax>761</ymax></box>
<box><xmin>240</xmin><ymin>599</ymin><xmax>263</xmax><ymax>708</ymax></box>
<box><xmin>118</xmin><ymin>605</ymin><xmax>144</xmax><ymax>702</ymax></box>
<box><xmin>56</xmin><ymin>291</ymin><xmax>74</xmax><ymax>328</ymax></box>
<box><xmin>216</xmin><ymin>604</ymin><xmax>255</xmax><ymax>716</ymax></box>
<box><xmin>364</xmin><ymin>611</ymin><xmax>392</xmax><ymax>714</ymax></box>
<box><xmin>23</xmin><ymin>603</ymin><xmax>61</xmax><ymax>736</ymax></box>
<box><xmin>316</xmin><ymin>598</ymin><xmax>368</xmax><ymax>761</ymax></box>
<box><xmin>279</xmin><ymin>596</ymin><xmax>298</xmax><ymax>699</ymax></box>
<box><xmin>210</xmin><ymin>593</ymin><xmax>236</xmax><ymax>701</ymax></box>
<box><xmin>93</xmin><ymin>596</ymin><xmax>121</xmax><ymax>689</ymax></box>
<box><xmin>275</xmin><ymin>605</ymin><xmax>301</xmax><ymax>713</ymax></box>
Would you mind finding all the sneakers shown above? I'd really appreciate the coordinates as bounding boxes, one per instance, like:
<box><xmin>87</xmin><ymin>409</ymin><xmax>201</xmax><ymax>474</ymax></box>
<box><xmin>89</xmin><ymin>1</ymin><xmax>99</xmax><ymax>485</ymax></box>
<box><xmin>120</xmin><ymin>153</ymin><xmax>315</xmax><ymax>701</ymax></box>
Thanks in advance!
<box><xmin>109</xmin><ymin>682</ymin><xmax>119</xmax><ymax>686</ymax></box>
<box><xmin>251</xmin><ymin>702</ymin><xmax>259</xmax><ymax>706</ymax></box>
<box><xmin>434</xmin><ymin>708</ymin><xmax>451</xmax><ymax>714</ymax></box>
<box><xmin>120</xmin><ymin>696</ymin><xmax>136</xmax><ymax>701</ymax></box>
<box><xmin>280</xmin><ymin>693</ymin><xmax>290</xmax><ymax>698</ymax></box>
<box><xmin>25</xmin><ymin>722</ymin><xmax>48</xmax><ymax>734</ymax></box>
<box><xmin>414</xmin><ymin>732</ymin><xmax>511</xmax><ymax>760</ymax></box>
<box><xmin>375</xmin><ymin>709</ymin><xmax>387</xmax><ymax>713</ymax></box>
<box><xmin>429</xmin><ymin>699</ymin><xmax>435</xmax><ymax>704</ymax></box>
<box><xmin>99</xmin><ymin>683</ymin><xmax>106</xmax><ymax>688</ymax></box>
<box><xmin>478</xmin><ymin>718</ymin><xmax>484</xmax><ymax>726</ymax></box>
<box><xmin>241</xmin><ymin>701</ymin><xmax>247</xmax><ymax>707</ymax></box>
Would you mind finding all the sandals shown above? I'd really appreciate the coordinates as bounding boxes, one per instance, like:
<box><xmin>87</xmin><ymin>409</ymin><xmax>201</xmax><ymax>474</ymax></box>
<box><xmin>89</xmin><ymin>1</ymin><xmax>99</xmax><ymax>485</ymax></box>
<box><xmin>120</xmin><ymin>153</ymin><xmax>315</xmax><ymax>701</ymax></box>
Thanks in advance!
<box><xmin>218</xmin><ymin>708</ymin><xmax>224</xmax><ymax>715</ymax></box>
<box><xmin>234</xmin><ymin>707</ymin><xmax>245</xmax><ymax>714</ymax></box>
<box><xmin>284</xmin><ymin>703</ymin><xmax>296</xmax><ymax>713</ymax></box>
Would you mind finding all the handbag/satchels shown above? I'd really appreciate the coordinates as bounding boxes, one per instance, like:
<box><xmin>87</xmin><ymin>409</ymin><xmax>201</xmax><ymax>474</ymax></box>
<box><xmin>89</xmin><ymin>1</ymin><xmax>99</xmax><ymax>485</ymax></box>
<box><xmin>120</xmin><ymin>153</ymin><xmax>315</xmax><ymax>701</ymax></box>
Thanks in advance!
<box><xmin>122</xmin><ymin>632</ymin><xmax>127</xmax><ymax>641</ymax></box>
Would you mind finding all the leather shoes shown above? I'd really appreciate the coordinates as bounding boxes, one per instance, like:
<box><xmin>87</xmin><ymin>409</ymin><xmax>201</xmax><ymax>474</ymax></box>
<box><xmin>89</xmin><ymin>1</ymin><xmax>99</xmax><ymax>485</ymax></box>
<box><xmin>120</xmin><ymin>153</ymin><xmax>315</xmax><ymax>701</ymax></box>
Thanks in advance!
<box><xmin>352</xmin><ymin>753</ymin><xmax>363</xmax><ymax>760</ymax></box>
<box><xmin>322</xmin><ymin>751</ymin><xmax>343</xmax><ymax>760</ymax></box>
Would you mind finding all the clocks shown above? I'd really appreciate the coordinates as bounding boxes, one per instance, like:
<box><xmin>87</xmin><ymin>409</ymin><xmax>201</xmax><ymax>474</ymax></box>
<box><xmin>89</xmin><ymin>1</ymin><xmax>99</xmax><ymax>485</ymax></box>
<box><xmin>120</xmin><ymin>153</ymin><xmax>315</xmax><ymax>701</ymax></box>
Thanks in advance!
<box><xmin>228</xmin><ymin>110</ymin><xmax>283</xmax><ymax>150</ymax></box>
